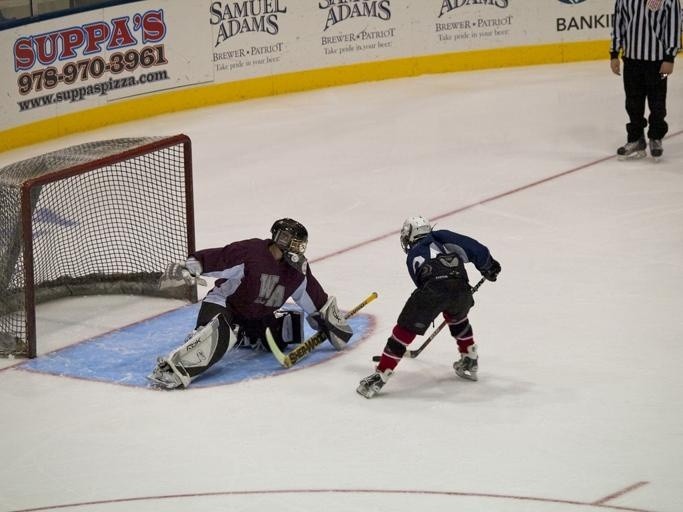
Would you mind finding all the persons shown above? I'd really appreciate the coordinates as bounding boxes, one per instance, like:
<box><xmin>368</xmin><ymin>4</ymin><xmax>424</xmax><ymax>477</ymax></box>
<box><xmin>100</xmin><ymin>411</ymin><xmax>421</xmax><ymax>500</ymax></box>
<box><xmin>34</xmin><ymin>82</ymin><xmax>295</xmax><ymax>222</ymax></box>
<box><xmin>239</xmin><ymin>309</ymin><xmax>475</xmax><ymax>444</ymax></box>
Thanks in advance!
<box><xmin>608</xmin><ymin>0</ymin><xmax>682</xmax><ymax>165</ymax></box>
<box><xmin>354</xmin><ymin>212</ymin><xmax>502</xmax><ymax>400</ymax></box>
<box><xmin>146</xmin><ymin>214</ymin><xmax>354</xmax><ymax>389</ymax></box>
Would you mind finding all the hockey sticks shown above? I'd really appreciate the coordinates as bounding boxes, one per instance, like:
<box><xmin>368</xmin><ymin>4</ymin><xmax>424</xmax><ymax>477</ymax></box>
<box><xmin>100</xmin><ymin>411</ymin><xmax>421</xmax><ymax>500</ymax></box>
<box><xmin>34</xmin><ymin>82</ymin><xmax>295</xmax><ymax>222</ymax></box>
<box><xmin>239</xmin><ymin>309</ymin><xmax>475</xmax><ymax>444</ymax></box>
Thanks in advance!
<box><xmin>389</xmin><ymin>277</ymin><xmax>486</xmax><ymax>359</ymax></box>
<box><xmin>266</xmin><ymin>292</ymin><xmax>377</xmax><ymax>368</ymax></box>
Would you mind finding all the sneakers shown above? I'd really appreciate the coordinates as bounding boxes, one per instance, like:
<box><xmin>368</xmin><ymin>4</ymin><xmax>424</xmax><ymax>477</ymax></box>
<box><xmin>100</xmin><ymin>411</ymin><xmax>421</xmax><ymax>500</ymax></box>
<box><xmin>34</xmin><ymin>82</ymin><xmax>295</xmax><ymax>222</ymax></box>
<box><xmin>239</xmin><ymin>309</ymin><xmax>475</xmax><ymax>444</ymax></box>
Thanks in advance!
<box><xmin>150</xmin><ymin>362</ymin><xmax>178</xmax><ymax>388</ymax></box>
<box><xmin>617</xmin><ymin>139</ymin><xmax>647</xmax><ymax>159</ymax></box>
<box><xmin>649</xmin><ymin>139</ymin><xmax>662</xmax><ymax>162</ymax></box>
<box><xmin>453</xmin><ymin>356</ymin><xmax>478</xmax><ymax>382</ymax></box>
<box><xmin>354</xmin><ymin>374</ymin><xmax>384</xmax><ymax>400</ymax></box>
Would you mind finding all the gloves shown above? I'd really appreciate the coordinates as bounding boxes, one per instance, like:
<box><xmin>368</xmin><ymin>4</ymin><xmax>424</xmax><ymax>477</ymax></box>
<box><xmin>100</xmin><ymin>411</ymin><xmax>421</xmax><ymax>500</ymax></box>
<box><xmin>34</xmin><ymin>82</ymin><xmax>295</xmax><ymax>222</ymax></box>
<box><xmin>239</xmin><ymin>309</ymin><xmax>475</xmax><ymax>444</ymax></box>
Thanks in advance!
<box><xmin>479</xmin><ymin>256</ymin><xmax>500</xmax><ymax>281</ymax></box>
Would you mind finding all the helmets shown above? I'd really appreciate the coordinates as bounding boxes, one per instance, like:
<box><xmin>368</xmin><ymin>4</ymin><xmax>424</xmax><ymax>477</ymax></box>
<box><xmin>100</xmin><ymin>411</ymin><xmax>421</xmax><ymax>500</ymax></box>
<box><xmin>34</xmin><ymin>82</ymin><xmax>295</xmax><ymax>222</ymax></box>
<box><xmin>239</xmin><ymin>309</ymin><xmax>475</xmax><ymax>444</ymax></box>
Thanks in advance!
<box><xmin>400</xmin><ymin>216</ymin><xmax>432</xmax><ymax>252</ymax></box>
<box><xmin>271</xmin><ymin>218</ymin><xmax>308</xmax><ymax>263</ymax></box>
